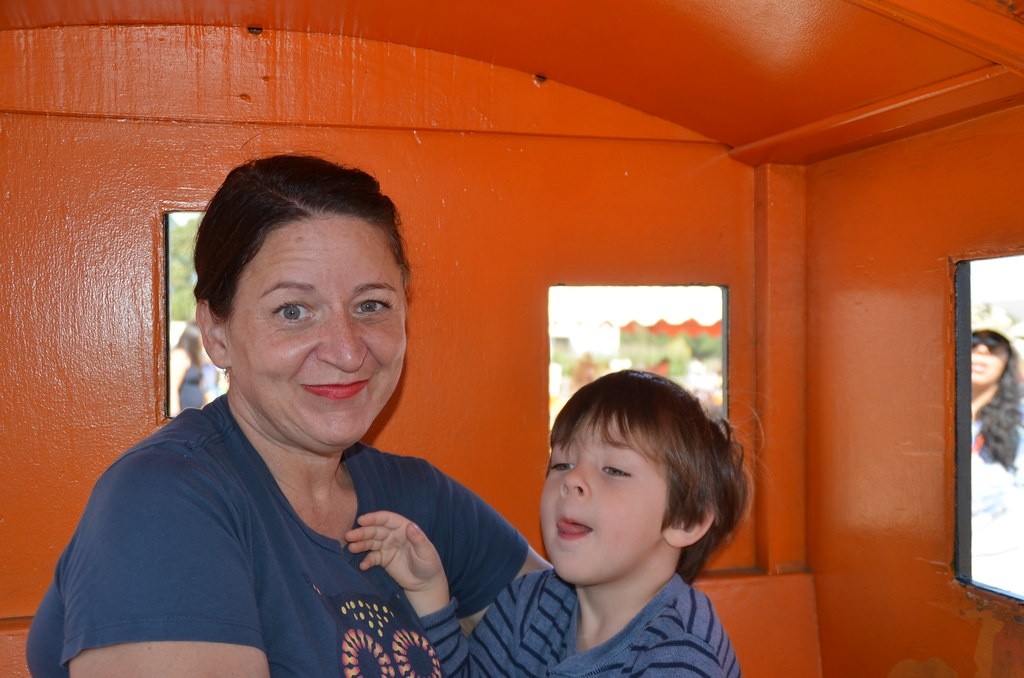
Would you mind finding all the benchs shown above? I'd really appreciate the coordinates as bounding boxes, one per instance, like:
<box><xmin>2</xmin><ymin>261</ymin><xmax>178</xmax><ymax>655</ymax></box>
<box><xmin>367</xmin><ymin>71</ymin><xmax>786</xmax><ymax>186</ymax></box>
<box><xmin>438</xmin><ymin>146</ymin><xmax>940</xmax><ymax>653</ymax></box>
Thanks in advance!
<box><xmin>0</xmin><ymin>566</ymin><xmax>819</xmax><ymax>678</ymax></box>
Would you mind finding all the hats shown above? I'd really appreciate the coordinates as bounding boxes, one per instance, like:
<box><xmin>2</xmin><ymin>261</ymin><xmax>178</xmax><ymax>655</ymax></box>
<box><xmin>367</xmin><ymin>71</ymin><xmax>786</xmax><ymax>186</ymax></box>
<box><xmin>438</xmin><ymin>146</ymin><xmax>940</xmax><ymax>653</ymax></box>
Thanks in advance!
<box><xmin>969</xmin><ymin>308</ymin><xmax>1019</xmax><ymax>352</ymax></box>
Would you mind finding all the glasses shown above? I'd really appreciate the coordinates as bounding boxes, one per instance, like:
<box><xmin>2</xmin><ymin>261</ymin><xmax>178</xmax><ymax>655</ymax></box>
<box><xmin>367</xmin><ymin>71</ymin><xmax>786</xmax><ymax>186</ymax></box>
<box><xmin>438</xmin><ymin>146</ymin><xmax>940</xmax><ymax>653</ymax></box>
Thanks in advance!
<box><xmin>970</xmin><ymin>331</ymin><xmax>1004</xmax><ymax>354</ymax></box>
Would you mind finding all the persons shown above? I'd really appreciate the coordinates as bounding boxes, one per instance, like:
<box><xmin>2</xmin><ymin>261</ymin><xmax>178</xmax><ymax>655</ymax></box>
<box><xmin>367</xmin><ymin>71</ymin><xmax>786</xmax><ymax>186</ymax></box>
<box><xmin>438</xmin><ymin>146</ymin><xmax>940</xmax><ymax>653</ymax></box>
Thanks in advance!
<box><xmin>346</xmin><ymin>370</ymin><xmax>753</xmax><ymax>678</ymax></box>
<box><xmin>969</xmin><ymin>317</ymin><xmax>1024</xmax><ymax>595</ymax></box>
<box><xmin>26</xmin><ymin>156</ymin><xmax>553</xmax><ymax>678</ymax></box>
<box><xmin>655</xmin><ymin>358</ymin><xmax>670</xmax><ymax>375</ymax></box>
<box><xmin>572</xmin><ymin>353</ymin><xmax>596</xmax><ymax>388</ymax></box>
<box><xmin>177</xmin><ymin>321</ymin><xmax>219</xmax><ymax>413</ymax></box>
